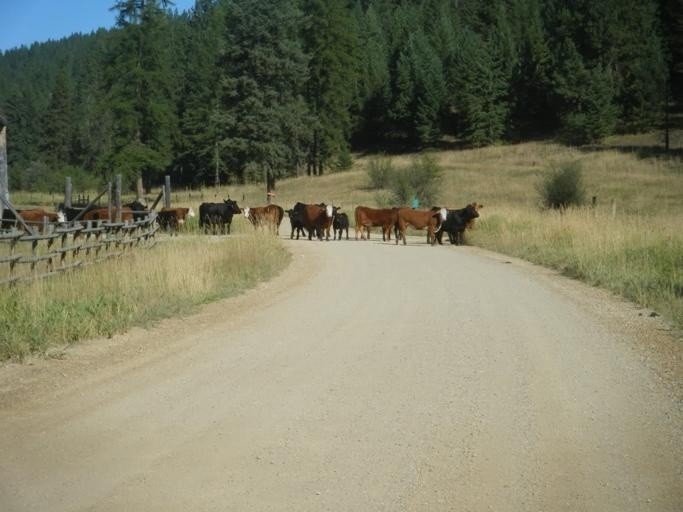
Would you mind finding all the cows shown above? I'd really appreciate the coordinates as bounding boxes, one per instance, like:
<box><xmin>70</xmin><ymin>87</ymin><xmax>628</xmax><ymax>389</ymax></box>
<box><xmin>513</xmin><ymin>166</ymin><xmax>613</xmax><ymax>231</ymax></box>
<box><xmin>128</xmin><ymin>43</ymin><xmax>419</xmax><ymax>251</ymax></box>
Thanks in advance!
<box><xmin>199</xmin><ymin>199</ymin><xmax>241</xmax><ymax>235</ymax></box>
<box><xmin>240</xmin><ymin>205</ymin><xmax>284</xmax><ymax>235</ymax></box>
<box><xmin>285</xmin><ymin>202</ymin><xmax>348</xmax><ymax>241</ymax></box>
<box><xmin>355</xmin><ymin>202</ymin><xmax>483</xmax><ymax>246</ymax></box>
<box><xmin>157</xmin><ymin>207</ymin><xmax>195</xmax><ymax>239</ymax></box>
<box><xmin>2</xmin><ymin>202</ymin><xmax>148</xmax><ymax>237</ymax></box>
